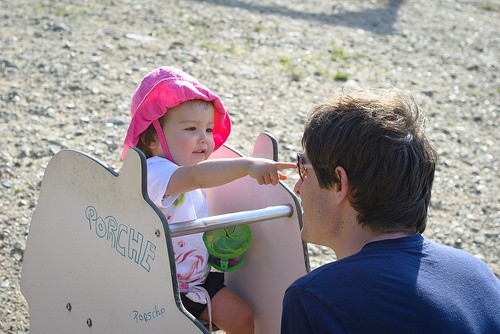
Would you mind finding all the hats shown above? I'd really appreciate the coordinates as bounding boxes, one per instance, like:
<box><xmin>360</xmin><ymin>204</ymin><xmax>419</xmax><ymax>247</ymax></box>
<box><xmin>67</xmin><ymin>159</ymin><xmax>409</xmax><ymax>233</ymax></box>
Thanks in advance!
<box><xmin>121</xmin><ymin>66</ymin><xmax>232</xmax><ymax>163</ymax></box>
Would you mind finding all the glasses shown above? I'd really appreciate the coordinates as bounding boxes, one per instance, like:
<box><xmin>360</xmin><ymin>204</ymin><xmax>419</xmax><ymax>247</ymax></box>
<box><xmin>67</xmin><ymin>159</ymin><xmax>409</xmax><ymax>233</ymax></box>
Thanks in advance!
<box><xmin>296</xmin><ymin>153</ymin><xmax>328</xmax><ymax>182</ymax></box>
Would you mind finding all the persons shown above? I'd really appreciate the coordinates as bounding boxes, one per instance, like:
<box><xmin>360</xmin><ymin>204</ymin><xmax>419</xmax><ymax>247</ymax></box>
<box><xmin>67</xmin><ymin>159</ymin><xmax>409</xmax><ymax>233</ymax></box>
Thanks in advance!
<box><xmin>120</xmin><ymin>64</ymin><xmax>299</xmax><ymax>334</ymax></box>
<box><xmin>279</xmin><ymin>90</ymin><xmax>500</xmax><ymax>334</ymax></box>
